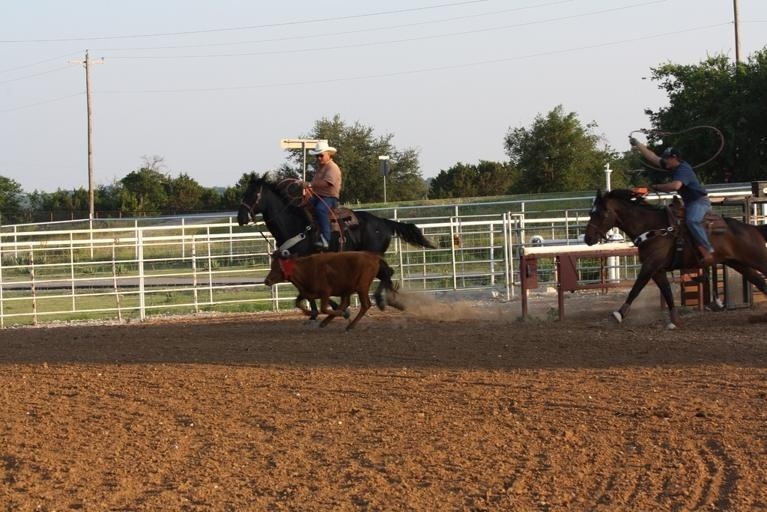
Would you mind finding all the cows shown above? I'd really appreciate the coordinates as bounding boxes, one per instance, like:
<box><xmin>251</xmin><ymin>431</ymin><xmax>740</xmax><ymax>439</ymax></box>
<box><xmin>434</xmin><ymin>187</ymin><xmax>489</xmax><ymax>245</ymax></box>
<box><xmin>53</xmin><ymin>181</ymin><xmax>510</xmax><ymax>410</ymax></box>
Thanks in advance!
<box><xmin>264</xmin><ymin>250</ymin><xmax>401</xmax><ymax>331</ymax></box>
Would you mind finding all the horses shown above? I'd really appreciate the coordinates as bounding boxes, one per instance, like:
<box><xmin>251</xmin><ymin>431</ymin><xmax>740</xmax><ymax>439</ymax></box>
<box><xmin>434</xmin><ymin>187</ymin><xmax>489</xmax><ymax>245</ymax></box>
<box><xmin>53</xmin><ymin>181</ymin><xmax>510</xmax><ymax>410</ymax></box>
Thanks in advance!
<box><xmin>237</xmin><ymin>172</ymin><xmax>438</xmax><ymax>319</ymax></box>
<box><xmin>583</xmin><ymin>187</ymin><xmax>767</xmax><ymax>330</ymax></box>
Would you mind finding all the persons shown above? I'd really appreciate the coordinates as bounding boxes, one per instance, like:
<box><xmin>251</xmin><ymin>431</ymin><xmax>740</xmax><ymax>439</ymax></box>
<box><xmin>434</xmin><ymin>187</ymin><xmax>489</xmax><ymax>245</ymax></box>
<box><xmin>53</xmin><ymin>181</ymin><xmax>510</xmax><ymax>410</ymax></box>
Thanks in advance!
<box><xmin>292</xmin><ymin>139</ymin><xmax>342</xmax><ymax>252</ymax></box>
<box><xmin>628</xmin><ymin>134</ymin><xmax>717</xmax><ymax>265</ymax></box>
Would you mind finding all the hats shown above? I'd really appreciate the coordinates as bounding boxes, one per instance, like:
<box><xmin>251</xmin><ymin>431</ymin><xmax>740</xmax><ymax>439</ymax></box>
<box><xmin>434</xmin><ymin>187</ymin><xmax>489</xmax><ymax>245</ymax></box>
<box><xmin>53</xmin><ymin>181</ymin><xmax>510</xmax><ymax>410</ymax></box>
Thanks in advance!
<box><xmin>309</xmin><ymin>142</ymin><xmax>337</xmax><ymax>155</ymax></box>
<box><xmin>661</xmin><ymin>148</ymin><xmax>680</xmax><ymax>158</ymax></box>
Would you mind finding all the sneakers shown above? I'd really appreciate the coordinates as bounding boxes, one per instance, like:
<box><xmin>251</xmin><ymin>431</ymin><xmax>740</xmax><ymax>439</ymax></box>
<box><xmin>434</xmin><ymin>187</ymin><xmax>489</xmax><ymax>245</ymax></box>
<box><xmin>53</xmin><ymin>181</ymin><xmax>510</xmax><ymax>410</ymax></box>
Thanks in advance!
<box><xmin>699</xmin><ymin>248</ymin><xmax>714</xmax><ymax>262</ymax></box>
<box><xmin>315</xmin><ymin>242</ymin><xmax>329</xmax><ymax>249</ymax></box>
